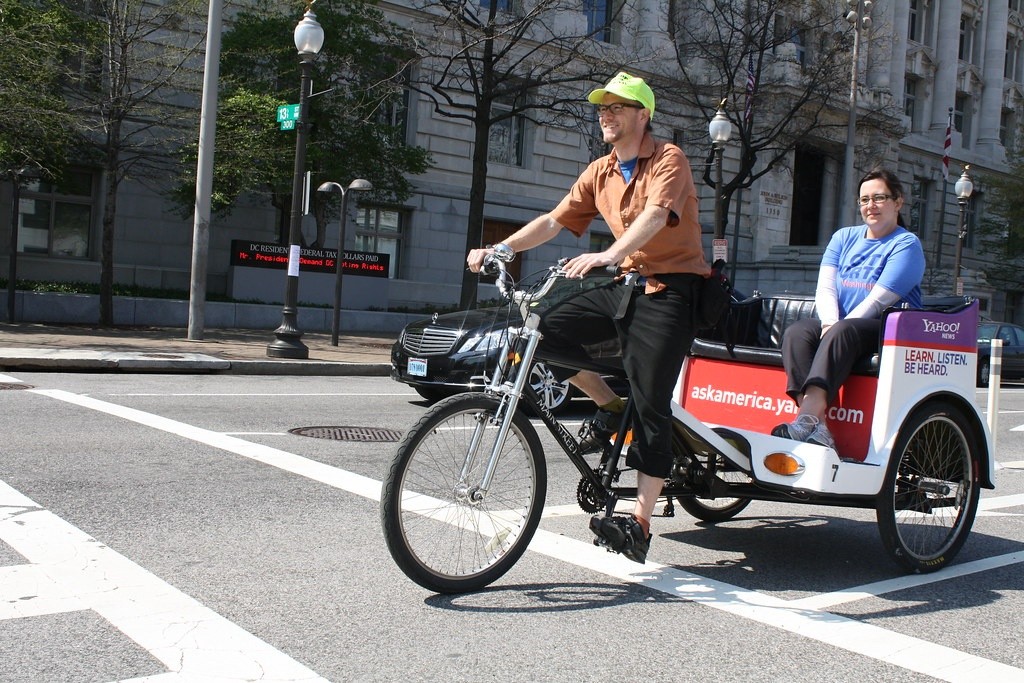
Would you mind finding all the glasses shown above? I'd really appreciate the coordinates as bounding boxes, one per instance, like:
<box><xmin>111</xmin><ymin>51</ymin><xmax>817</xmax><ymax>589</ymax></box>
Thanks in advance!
<box><xmin>596</xmin><ymin>102</ymin><xmax>644</xmax><ymax>116</ymax></box>
<box><xmin>856</xmin><ymin>193</ymin><xmax>897</xmax><ymax>206</ymax></box>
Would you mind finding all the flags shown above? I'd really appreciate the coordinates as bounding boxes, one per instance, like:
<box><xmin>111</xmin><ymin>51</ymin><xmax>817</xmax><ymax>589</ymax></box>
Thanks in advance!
<box><xmin>942</xmin><ymin>115</ymin><xmax>952</xmax><ymax>182</ymax></box>
<box><xmin>744</xmin><ymin>52</ymin><xmax>755</xmax><ymax>119</ymax></box>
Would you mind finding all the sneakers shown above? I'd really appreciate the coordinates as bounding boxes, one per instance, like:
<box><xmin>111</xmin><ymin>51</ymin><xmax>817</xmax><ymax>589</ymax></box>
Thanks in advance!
<box><xmin>805</xmin><ymin>425</ymin><xmax>840</xmax><ymax>457</ymax></box>
<box><xmin>771</xmin><ymin>413</ymin><xmax>820</xmax><ymax>441</ymax></box>
<box><xmin>589</xmin><ymin>515</ymin><xmax>652</xmax><ymax>564</ymax></box>
<box><xmin>574</xmin><ymin>406</ymin><xmax>638</xmax><ymax>455</ymax></box>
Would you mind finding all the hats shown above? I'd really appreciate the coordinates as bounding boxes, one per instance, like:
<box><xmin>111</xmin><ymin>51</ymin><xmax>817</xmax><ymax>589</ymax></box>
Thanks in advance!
<box><xmin>588</xmin><ymin>71</ymin><xmax>656</xmax><ymax>122</ymax></box>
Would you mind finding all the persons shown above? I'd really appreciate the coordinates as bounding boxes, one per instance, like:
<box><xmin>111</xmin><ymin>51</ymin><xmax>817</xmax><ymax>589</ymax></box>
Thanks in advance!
<box><xmin>467</xmin><ymin>72</ymin><xmax>710</xmax><ymax>564</ymax></box>
<box><xmin>770</xmin><ymin>167</ymin><xmax>925</xmax><ymax>456</ymax></box>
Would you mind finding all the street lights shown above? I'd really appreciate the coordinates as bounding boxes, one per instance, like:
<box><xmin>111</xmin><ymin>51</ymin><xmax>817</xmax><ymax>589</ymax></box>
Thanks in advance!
<box><xmin>0</xmin><ymin>166</ymin><xmax>41</xmax><ymax>323</ymax></box>
<box><xmin>708</xmin><ymin>99</ymin><xmax>732</xmax><ymax>277</ymax></box>
<box><xmin>952</xmin><ymin>165</ymin><xmax>974</xmax><ymax>297</ymax></box>
<box><xmin>266</xmin><ymin>0</ymin><xmax>324</xmax><ymax>360</ymax></box>
<box><xmin>844</xmin><ymin>0</ymin><xmax>873</xmax><ymax>229</ymax></box>
<box><xmin>317</xmin><ymin>179</ymin><xmax>373</xmax><ymax>346</ymax></box>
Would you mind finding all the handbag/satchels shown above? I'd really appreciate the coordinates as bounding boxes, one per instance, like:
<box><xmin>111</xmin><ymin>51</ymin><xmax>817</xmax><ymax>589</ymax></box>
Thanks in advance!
<box><xmin>697</xmin><ymin>258</ymin><xmax>737</xmax><ymax>360</ymax></box>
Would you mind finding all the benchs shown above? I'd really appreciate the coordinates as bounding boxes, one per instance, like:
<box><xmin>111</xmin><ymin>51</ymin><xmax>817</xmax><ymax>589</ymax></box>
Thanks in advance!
<box><xmin>682</xmin><ymin>291</ymin><xmax>975</xmax><ymax>462</ymax></box>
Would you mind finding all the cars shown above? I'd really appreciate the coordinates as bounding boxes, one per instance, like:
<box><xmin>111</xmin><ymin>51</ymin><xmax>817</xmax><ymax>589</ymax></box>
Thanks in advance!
<box><xmin>975</xmin><ymin>322</ymin><xmax>1024</xmax><ymax>389</ymax></box>
<box><xmin>388</xmin><ymin>277</ymin><xmax>747</xmax><ymax>417</ymax></box>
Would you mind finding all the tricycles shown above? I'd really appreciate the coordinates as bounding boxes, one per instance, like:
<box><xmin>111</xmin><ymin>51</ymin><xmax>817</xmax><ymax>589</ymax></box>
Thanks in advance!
<box><xmin>379</xmin><ymin>253</ymin><xmax>1004</xmax><ymax>595</ymax></box>
